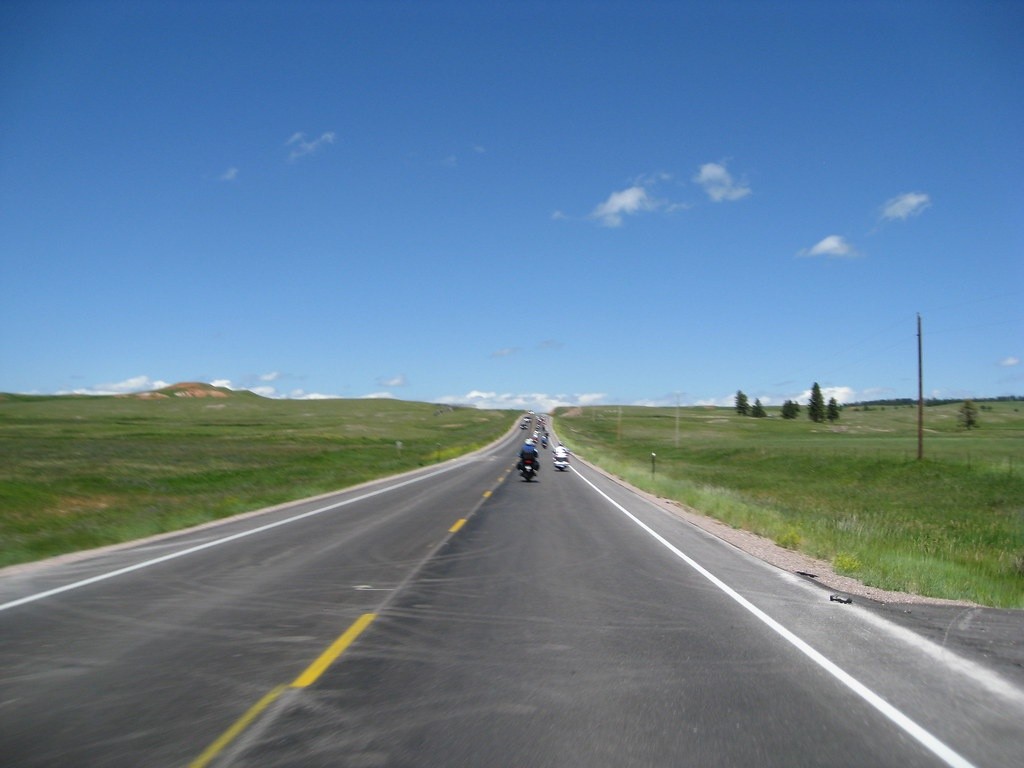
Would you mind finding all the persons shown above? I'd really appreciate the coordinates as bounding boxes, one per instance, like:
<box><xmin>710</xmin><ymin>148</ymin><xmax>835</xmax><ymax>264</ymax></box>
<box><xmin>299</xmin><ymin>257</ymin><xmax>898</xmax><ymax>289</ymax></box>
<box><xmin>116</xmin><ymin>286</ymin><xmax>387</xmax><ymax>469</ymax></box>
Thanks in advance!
<box><xmin>552</xmin><ymin>441</ymin><xmax>570</xmax><ymax>470</ymax></box>
<box><xmin>516</xmin><ymin>437</ymin><xmax>540</xmax><ymax>475</ymax></box>
<box><xmin>532</xmin><ymin>414</ymin><xmax>549</xmax><ymax>448</ymax></box>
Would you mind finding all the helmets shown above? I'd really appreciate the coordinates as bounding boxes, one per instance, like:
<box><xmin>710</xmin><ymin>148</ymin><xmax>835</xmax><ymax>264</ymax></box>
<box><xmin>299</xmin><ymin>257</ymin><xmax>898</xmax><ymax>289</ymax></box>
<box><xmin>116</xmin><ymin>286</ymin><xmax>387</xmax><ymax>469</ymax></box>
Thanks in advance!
<box><xmin>525</xmin><ymin>438</ymin><xmax>533</xmax><ymax>445</ymax></box>
<box><xmin>559</xmin><ymin>441</ymin><xmax>563</xmax><ymax>445</ymax></box>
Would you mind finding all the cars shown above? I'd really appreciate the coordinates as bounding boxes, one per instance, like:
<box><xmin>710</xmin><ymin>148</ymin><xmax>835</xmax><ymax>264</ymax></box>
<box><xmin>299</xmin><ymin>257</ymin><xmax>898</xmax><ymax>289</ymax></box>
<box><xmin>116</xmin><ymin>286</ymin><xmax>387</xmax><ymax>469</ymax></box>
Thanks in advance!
<box><xmin>528</xmin><ymin>410</ymin><xmax>535</xmax><ymax>415</ymax></box>
<box><xmin>520</xmin><ymin>424</ymin><xmax>528</xmax><ymax>430</ymax></box>
<box><xmin>524</xmin><ymin>416</ymin><xmax>532</xmax><ymax>422</ymax></box>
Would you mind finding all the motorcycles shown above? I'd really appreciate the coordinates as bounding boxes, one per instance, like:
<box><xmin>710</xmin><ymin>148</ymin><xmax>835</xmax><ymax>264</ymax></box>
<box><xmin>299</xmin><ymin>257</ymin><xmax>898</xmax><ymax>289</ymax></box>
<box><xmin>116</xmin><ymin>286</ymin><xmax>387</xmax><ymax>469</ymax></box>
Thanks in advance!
<box><xmin>553</xmin><ymin>450</ymin><xmax>570</xmax><ymax>471</ymax></box>
<box><xmin>517</xmin><ymin>452</ymin><xmax>538</xmax><ymax>482</ymax></box>
